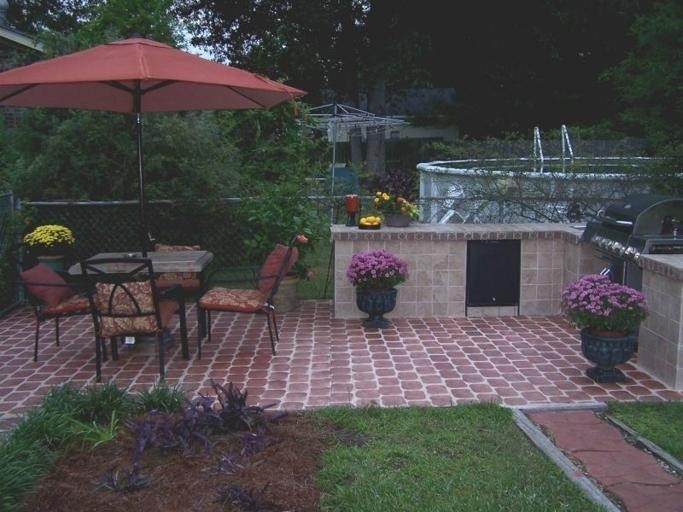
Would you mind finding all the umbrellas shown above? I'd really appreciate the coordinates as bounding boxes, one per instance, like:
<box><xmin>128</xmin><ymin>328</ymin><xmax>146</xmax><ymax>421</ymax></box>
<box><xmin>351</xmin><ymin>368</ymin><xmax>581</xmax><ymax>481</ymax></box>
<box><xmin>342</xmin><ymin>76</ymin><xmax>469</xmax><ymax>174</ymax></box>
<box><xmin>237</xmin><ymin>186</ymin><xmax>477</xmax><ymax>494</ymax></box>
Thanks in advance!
<box><xmin>0</xmin><ymin>34</ymin><xmax>312</xmax><ymax>258</ymax></box>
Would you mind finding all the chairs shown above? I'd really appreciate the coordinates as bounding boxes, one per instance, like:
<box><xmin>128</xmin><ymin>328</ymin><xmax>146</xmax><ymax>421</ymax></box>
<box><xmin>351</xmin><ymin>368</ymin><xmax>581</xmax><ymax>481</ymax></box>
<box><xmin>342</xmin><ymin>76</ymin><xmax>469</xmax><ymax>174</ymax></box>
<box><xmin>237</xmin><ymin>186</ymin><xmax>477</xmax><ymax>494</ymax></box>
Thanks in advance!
<box><xmin>147</xmin><ymin>234</ymin><xmax>211</xmax><ymax>342</ymax></box>
<box><xmin>3</xmin><ymin>241</ymin><xmax>110</xmax><ymax>361</ymax></box>
<box><xmin>197</xmin><ymin>235</ymin><xmax>305</xmax><ymax>355</ymax></box>
<box><xmin>77</xmin><ymin>254</ymin><xmax>192</xmax><ymax>385</ymax></box>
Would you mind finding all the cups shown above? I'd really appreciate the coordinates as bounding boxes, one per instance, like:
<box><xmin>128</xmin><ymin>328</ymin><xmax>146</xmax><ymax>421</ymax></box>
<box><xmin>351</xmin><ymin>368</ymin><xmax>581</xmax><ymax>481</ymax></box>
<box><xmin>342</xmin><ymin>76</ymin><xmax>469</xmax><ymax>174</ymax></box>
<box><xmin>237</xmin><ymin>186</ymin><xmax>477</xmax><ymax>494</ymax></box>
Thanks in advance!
<box><xmin>124</xmin><ymin>252</ymin><xmax>137</xmax><ymax>261</ymax></box>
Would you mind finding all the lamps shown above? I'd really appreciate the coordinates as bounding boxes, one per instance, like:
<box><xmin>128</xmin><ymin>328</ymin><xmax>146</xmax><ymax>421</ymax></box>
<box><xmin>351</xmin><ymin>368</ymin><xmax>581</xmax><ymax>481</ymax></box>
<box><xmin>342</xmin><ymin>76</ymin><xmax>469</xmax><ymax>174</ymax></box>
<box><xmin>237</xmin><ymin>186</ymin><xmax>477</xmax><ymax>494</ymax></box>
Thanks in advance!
<box><xmin>341</xmin><ymin>192</ymin><xmax>360</xmax><ymax>226</ymax></box>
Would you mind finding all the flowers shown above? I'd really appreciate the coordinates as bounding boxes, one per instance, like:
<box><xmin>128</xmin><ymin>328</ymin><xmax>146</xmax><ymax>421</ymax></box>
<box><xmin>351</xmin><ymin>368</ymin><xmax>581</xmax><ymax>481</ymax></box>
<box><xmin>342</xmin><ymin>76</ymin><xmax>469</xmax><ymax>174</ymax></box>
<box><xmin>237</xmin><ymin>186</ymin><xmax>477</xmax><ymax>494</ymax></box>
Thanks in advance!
<box><xmin>560</xmin><ymin>273</ymin><xmax>655</xmax><ymax>334</ymax></box>
<box><xmin>346</xmin><ymin>252</ymin><xmax>407</xmax><ymax>288</ymax></box>
<box><xmin>21</xmin><ymin>223</ymin><xmax>74</xmax><ymax>255</ymax></box>
<box><xmin>271</xmin><ymin>233</ymin><xmax>317</xmax><ymax>280</ymax></box>
<box><xmin>359</xmin><ymin>215</ymin><xmax>379</xmax><ymax>225</ymax></box>
<box><xmin>373</xmin><ymin>190</ymin><xmax>422</xmax><ymax>215</ymax></box>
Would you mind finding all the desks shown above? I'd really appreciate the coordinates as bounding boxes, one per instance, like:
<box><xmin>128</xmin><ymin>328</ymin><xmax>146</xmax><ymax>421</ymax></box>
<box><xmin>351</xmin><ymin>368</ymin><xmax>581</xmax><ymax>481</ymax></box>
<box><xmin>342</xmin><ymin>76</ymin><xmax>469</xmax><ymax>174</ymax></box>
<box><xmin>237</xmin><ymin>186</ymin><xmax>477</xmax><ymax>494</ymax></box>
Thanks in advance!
<box><xmin>66</xmin><ymin>247</ymin><xmax>215</xmax><ymax>361</ymax></box>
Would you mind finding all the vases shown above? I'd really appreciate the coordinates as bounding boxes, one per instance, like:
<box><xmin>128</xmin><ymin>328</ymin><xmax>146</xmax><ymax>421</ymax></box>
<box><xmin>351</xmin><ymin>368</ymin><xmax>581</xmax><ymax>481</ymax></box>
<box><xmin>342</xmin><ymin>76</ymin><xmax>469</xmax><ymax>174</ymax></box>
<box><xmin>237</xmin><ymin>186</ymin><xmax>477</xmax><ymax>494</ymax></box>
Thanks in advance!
<box><xmin>574</xmin><ymin>325</ymin><xmax>642</xmax><ymax>386</ymax></box>
<box><xmin>32</xmin><ymin>253</ymin><xmax>64</xmax><ymax>272</ymax></box>
<box><xmin>258</xmin><ymin>273</ymin><xmax>298</xmax><ymax>313</ymax></box>
<box><xmin>355</xmin><ymin>287</ymin><xmax>397</xmax><ymax>328</ymax></box>
<box><xmin>382</xmin><ymin>214</ymin><xmax>412</xmax><ymax>227</ymax></box>
